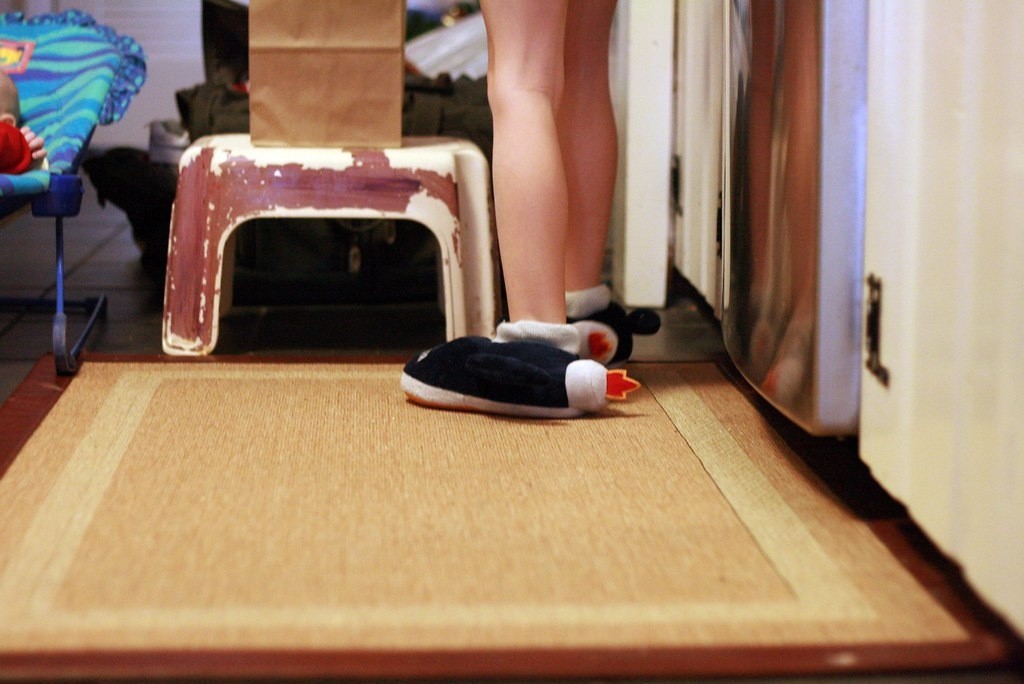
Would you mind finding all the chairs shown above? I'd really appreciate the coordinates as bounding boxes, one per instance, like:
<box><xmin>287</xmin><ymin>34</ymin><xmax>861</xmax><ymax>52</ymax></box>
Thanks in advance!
<box><xmin>0</xmin><ymin>8</ymin><xmax>147</xmax><ymax>376</ymax></box>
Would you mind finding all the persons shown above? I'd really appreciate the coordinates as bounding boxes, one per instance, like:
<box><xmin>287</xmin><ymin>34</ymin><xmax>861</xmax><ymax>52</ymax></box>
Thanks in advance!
<box><xmin>744</xmin><ymin>0</ymin><xmax>820</xmax><ymax>399</ymax></box>
<box><xmin>0</xmin><ymin>71</ymin><xmax>48</xmax><ymax>171</ymax></box>
<box><xmin>402</xmin><ymin>0</ymin><xmax>661</xmax><ymax>417</ymax></box>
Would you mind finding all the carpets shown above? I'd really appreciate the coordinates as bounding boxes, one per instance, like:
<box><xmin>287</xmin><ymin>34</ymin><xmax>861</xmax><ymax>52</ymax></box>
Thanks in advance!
<box><xmin>0</xmin><ymin>354</ymin><xmax>1002</xmax><ymax>684</ymax></box>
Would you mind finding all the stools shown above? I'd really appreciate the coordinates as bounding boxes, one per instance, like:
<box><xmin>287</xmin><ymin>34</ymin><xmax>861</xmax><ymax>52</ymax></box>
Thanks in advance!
<box><xmin>161</xmin><ymin>134</ymin><xmax>501</xmax><ymax>356</ymax></box>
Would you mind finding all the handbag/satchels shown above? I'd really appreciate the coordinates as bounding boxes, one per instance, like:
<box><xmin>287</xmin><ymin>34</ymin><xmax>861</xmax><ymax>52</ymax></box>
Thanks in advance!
<box><xmin>176</xmin><ymin>64</ymin><xmax>504</xmax><ymax>306</ymax></box>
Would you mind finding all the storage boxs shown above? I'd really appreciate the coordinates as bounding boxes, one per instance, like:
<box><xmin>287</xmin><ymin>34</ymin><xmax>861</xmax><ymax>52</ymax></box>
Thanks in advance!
<box><xmin>248</xmin><ymin>0</ymin><xmax>406</xmax><ymax>149</ymax></box>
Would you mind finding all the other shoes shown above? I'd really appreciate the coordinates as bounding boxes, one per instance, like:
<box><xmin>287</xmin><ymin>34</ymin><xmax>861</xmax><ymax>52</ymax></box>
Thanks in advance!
<box><xmin>495</xmin><ymin>285</ymin><xmax>660</xmax><ymax>370</ymax></box>
<box><xmin>400</xmin><ymin>321</ymin><xmax>642</xmax><ymax>418</ymax></box>
